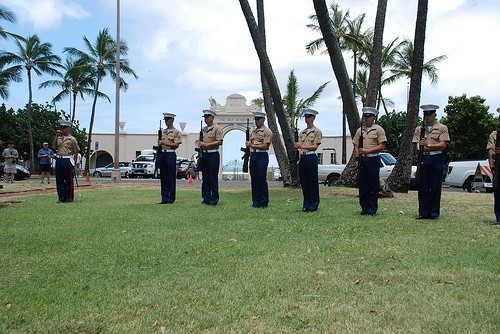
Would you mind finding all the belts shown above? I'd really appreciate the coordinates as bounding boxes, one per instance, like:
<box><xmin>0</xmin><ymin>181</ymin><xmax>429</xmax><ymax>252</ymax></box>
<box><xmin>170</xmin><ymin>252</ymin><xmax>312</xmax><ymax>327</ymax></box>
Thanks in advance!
<box><xmin>362</xmin><ymin>152</ymin><xmax>380</xmax><ymax>157</ymax></box>
<box><xmin>423</xmin><ymin>150</ymin><xmax>442</xmax><ymax>156</ymax></box>
<box><xmin>162</xmin><ymin>149</ymin><xmax>176</xmax><ymax>152</ymax></box>
<box><xmin>56</xmin><ymin>156</ymin><xmax>73</xmax><ymax>158</ymax></box>
<box><xmin>251</xmin><ymin>149</ymin><xmax>269</xmax><ymax>152</ymax></box>
<box><xmin>301</xmin><ymin>150</ymin><xmax>316</xmax><ymax>155</ymax></box>
<box><xmin>203</xmin><ymin>149</ymin><xmax>219</xmax><ymax>152</ymax></box>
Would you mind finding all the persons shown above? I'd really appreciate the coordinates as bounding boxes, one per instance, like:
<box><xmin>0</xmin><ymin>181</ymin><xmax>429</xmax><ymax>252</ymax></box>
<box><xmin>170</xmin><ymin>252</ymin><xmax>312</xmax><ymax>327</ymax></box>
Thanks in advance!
<box><xmin>246</xmin><ymin>112</ymin><xmax>274</xmax><ymax>209</ymax></box>
<box><xmin>412</xmin><ymin>104</ymin><xmax>450</xmax><ymax>219</ymax></box>
<box><xmin>486</xmin><ymin>107</ymin><xmax>500</xmax><ymax>225</ymax></box>
<box><xmin>38</xmin><ymin>141</ymin><xmax>54</xmax><ymax>184</ymax></box>
<box><xmin>52</xmin><ymin>121</ymin><xmax>80</xmax><ymax>203</ymax></box>
<box><xmin>352</xmin><ymin>107</ymin><xmax>388</xmax><ymax>216</ymax></box>
<box><xmin>157</xmin><ymin>112</ymin><xmax>182</xmax><ymax>205</ymax></box>
<box><xmin>195</xmin><ymin>110</ymin><xmax>223</xmax><ymax>207</ymax></box>
<box><xmin>294</xmin><ymin>107</ymin><xmax>322</xmax><ymax>212</ymax></box>
<box><xmin>2</xmin><ymin>142</ymin><xmax>19</xmax><ymax>184</ymax></box>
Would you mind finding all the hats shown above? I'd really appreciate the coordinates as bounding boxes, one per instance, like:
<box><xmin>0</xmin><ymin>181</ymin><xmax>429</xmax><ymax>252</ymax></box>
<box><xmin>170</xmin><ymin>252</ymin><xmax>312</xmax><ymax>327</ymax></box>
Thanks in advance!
<box><xmin>43</xmin><ymin>142</ymin><xmax>48</xmax><ymax>145</ymax></box>
<box><xmin>420</xmin><ymin>104</ymin><xmax>439</xmax><ymax>115</ymax></box>
<box><xmin>162</xmin><ymin>112</ymin><xmax>176</xmax><ymax>120</ymax></box>
<box><xmin>253</xmin><ymin>112</ymin><xmax>265</xmax><ymax>120</ymax></box>
<box><xmin>362</xmin><ymin>107</ymin><xmax>379</xmax><ymax>117</ymax></box>
<box><xmin>303</xmin><ymin>109</ymin><xmax>319</xmax><ymax>117</ymax></box>
<box><xmin>202</xmin><ymin>110</ymin><xmax>216</xmax><ymax>117</ymax></box>
<box><xmin>60</xmin><ymin>121</ymin><xmax>72</xmax><ymax>129</ymax></box>
<box><xmin>496</xmin><ymin>105</ymin><xmax>500</xmax><ymax>113</ymax></box>
<box><xmin>8</xmin><ymin>141</ymin><xmax>14</xmax><ymax>144</ymax></box>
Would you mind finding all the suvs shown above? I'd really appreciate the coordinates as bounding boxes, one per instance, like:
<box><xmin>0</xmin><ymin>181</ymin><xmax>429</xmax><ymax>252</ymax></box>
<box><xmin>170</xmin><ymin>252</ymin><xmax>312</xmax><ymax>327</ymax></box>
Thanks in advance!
<box><xmin>128</xmin><ymin>150</ymin><xmax>161</xmax><ymax>179</ymax></box>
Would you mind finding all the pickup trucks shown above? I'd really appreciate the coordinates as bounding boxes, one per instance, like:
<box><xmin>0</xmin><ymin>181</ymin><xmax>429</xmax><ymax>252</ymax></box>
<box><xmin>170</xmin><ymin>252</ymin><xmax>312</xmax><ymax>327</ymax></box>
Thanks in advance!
<box><xmin>445</xmin><ymin>158</ymin><xmax>493</xmax><ymax>194</ymax></box>
<box><xmin>273</xmin><ymin>168</ymin><xmax>283</xmax><ymax>181</ymax></box>
<box><xmin>322</xmin><ymin>151</ymin><xmax>418</xmax><ymax>191</ymax></box>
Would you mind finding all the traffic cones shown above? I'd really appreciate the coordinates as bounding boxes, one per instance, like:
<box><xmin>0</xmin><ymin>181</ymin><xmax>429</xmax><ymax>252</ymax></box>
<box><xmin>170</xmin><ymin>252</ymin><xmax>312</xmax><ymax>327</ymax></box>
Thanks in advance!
<box><xmin>85</xmin><ymin>173</ymin><xmax>90</xmax><ymax>182</ymax></box>
<box><xmin>188</xmin><ymin>174</ymin><xmax>193</xmax><ymax>185</ymax></box>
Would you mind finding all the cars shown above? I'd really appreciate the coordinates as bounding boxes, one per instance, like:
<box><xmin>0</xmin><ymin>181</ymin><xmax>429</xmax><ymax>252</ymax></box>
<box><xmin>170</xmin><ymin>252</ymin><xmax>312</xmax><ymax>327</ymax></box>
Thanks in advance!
<box><xmin>175</xmin><ymin>157</ymin><xmax>199</xmax><ymax>181</ymax></box>
<box><xmin>89</xmin><ymin>161</ymin><xmax>132</xmax><ymax>178</ymax></box>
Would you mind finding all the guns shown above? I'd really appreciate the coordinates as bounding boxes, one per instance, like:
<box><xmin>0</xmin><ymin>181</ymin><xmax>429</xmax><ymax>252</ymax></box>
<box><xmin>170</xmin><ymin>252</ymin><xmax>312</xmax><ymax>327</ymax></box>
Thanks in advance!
<box><xmin>240</xmin><ymin>118</ymin><xmax>249</xmax><ymax>173</ymax></box>
<box><xmin>293</xmin><ymin>119</ymin><xmax>299</xmax><ymax>164</ymax></box>
<box><xmin>153</xmin><ymin>120</ymin><xmax>162</xmax><ymax>170</ymax></box>
<box><xmin>356</xmin><ymin>125</ymin><xmax>363</xmax><ymax>181</ymax></box>
<box><xmin>493</xmin><ymin>125</ymin><xmax>500</xmax><ymax>189</ymax></box>
<box><xmin>193</xmin><ymin>121</ymin><xmax>203</xmax><ymax>173</ymax></box>
<box><xmin>413</xmin><ymin>121</ymin><xmax>425</xmax><ymax>175</ymax></box>
